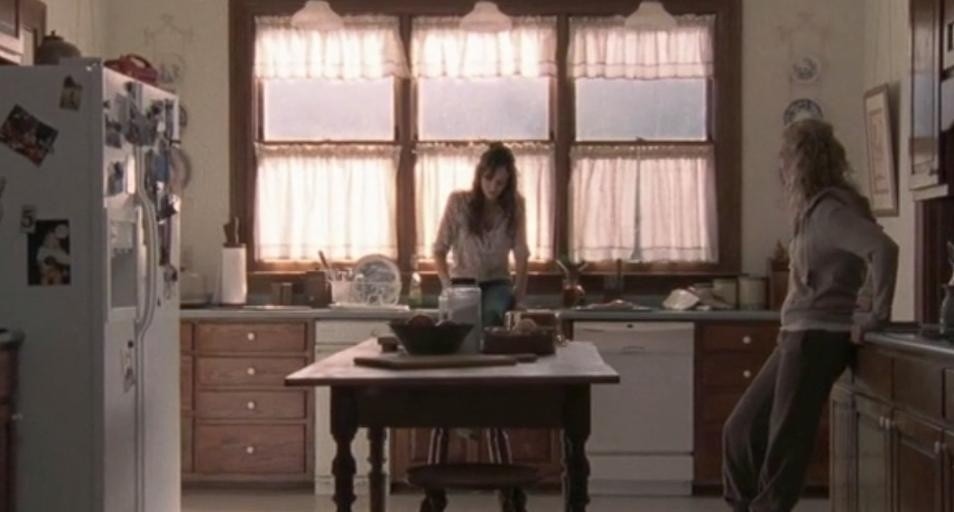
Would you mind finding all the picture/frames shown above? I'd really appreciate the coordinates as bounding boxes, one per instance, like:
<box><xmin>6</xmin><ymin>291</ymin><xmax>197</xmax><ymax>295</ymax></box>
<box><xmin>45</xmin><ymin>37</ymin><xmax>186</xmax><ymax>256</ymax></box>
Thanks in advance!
<box><xmin>861</xmin><ymin>0</ymin><xmax>954</xmax><ymax>219</ymax></box>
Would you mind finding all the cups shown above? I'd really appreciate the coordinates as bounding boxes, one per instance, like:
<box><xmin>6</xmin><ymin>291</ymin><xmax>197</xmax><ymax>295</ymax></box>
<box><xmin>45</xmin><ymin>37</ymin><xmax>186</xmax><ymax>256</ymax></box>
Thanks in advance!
<box><xmin>561</xmin><ymin>276</ymin><xmax>585</xmax><ymax>308</ymax></box>
<box><xmin>439</xmin><ymin>278</ymin><xmax>482</xmax><ymax>347</ymax></box>
<box><xmin>602</xmin><ymin>260</ymin><xmax>626</xmax><ymax>303</ymax></box>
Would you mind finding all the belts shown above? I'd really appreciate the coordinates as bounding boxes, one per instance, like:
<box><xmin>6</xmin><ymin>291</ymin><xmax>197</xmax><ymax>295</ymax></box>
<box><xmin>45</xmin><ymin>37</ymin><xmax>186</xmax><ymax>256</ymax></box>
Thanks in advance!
<box><xmin>452</xmin><ymin>277</ymin><xmax>510</xmax><ymax>289</ymax></box>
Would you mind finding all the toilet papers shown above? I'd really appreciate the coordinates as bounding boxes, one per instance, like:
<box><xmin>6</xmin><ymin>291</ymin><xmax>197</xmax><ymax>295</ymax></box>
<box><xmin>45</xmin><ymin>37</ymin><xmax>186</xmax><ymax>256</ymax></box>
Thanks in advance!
<box><xmin>219</xmin><ymin>244</ymin><xmax>249</xmax><ymax>307</ymax></box>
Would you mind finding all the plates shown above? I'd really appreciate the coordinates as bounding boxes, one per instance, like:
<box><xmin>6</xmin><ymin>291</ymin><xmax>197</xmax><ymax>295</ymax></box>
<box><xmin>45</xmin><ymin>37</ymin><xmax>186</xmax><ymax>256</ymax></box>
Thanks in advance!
<box><xmin>328</xmin><ymin>301</ymin><xmax>411</xmax><ymax>314</ymax></box>
<box><xmin>352</xmin><ymin>254</ymin><xmax>400</xmax><ymax>304</ymax></box>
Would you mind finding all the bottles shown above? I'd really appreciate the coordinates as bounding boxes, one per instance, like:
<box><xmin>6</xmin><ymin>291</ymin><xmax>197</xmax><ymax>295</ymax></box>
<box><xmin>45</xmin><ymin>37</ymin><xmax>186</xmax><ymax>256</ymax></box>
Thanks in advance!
<box><xmin>179</xmin><ymin>268</ymin><xmax>210</xmax><ymax>309</ymax></box>
<box><xmin>937</xmin><ymin>283</ymin><xmax>954</xmax><ymax>328</ymax></box>
<box><xmin>301</xmin><ymin>265</ymin><xmax>354</xmax><ymax>310</ymax></box>
<box><xmin>503</xmin><ymin>310</ymin><xmax>520</xmax><ymax>333</ymax></box>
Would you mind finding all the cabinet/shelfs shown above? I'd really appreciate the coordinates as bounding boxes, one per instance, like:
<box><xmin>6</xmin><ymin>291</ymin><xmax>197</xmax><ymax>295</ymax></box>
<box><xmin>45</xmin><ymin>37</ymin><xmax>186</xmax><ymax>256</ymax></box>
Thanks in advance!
<box><xmin>693</xmin><ymin>319</ymin><xmax>831</xmax><ymax>494</ymax></box>
<box><xmin>824</xmin><ymin>332</ymin><xmax>954</xmax><ymax>511</ymax></box>
<box><xmin>389</xmin><ymin>426</ymin><xmax>564</xmax><ymax>486</ymax></box>
<box><xmin>175</xmin><ymin>321</ymin><xmax>313</xmax><ymax>492</ymax></box>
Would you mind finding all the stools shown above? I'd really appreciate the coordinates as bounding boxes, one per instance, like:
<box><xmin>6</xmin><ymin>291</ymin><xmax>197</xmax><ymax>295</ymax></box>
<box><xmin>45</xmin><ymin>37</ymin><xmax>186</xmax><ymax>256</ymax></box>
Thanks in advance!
<box><xmin>402</xmin><ymin>461</ymin><xmax>541</xmax><ymax>512</ymax></box>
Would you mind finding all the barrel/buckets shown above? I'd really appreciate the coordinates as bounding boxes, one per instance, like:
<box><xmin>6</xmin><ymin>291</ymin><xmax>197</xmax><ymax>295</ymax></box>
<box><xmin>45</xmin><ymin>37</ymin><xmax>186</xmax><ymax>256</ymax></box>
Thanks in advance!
<box><xmin>712</xmin><ymin>279</ymin><xmax>738</xmax><ymax>305</ymax></box>
<box><xmin>435</xmin><ymin>275</ymin><xmax>485</xmax><ymax>327</ymax></box>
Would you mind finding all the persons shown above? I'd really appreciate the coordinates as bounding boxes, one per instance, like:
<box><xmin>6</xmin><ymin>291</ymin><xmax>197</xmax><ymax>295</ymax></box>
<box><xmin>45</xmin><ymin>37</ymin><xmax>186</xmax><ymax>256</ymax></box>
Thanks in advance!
<box><xmin>720</xmin><ymin>114</ymin><xmax>899</xmax><ymax>512</ymax></box>
<box><xmin>418</xmin><ymin>139</ymin><xmax>534</xmax><ymax>465</ymax></box>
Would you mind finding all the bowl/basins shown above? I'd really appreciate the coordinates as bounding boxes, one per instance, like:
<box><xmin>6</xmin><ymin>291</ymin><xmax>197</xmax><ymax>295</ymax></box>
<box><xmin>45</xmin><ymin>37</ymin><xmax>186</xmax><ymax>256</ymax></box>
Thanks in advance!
<box><xmin>483</xmin><ymin>323</ymin><xmax>547</xmax><ymax>353</ymax></box>
<box><xmin>386</xmin><ymin>322</ymin><xmax>473</xmax><ymax>355</ymax></box>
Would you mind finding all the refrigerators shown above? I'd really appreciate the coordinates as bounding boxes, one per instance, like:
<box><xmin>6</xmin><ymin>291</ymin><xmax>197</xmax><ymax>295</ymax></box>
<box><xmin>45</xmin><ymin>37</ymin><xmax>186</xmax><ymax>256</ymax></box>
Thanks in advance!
<box><xmin>1</xmin><ymin>59</ymin><xmax>184</xmax><ymax>512</ymax></box>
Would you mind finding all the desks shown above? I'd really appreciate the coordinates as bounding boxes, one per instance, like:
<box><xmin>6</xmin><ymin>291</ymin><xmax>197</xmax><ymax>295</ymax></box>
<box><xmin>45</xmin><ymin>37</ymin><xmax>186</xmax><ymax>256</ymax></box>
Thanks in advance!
<box><xmin>283</xmin><ymin>331</ymin><xmax>620</xmax><ymax>511</ymax></box>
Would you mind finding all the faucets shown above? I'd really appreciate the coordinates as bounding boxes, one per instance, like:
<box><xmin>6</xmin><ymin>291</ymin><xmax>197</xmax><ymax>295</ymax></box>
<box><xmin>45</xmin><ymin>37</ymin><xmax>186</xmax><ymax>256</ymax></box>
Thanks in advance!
<box><xmin>615</xmin><ymin>258</ymin><xmax>624</xmax><ymax>297</ymax></box>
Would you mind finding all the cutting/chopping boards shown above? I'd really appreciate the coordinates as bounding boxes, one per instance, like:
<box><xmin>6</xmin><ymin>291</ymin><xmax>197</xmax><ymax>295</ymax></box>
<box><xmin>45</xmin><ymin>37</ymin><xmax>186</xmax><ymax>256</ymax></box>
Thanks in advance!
<box><xmin>352</xmin><ymin>351</ymin><xmax>537</xmax><ymax>371</ymax></box>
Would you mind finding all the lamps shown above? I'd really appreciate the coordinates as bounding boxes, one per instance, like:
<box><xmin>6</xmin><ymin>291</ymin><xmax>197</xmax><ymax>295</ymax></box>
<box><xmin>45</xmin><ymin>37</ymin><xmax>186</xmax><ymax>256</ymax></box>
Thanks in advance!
<box><xmin>624</xmin><ymin>0</ymin><xmax>677</xmax><ymax>34</ymax></box>
<box><xmin>458</xmin><ymin>1</ymin><xmax>512</xmax><ymax>34</ymax></box>
<box><xmin>289</xmin><ymin>1</ymin><xmax>343</xmax><ymax>34</ymax></box>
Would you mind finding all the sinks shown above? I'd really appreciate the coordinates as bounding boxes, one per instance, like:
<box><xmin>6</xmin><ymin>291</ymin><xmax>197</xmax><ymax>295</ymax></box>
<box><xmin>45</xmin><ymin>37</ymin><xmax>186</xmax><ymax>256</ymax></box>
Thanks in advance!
<box><xmin>574</xmin><ymin>298</ymin><xmax>654</xmax><ymax>313</ymax></box>
